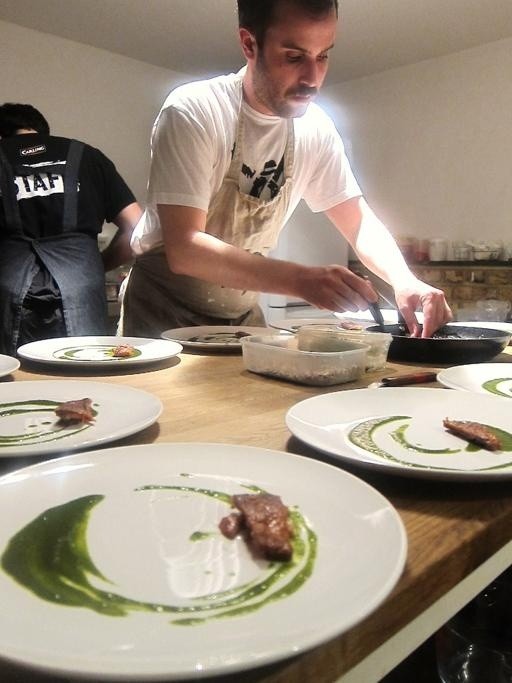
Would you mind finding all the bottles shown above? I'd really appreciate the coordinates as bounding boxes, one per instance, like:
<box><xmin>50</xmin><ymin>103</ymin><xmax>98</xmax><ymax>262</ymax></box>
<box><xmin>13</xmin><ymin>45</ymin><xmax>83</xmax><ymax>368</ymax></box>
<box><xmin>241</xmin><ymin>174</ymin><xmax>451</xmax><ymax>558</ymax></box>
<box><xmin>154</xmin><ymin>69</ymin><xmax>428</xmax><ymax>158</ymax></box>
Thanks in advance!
<box><xmin>397</xmin><ymin>236</ymin><xmax>447</xmax><ymax>262</ymax></box>
<box><xmin>450</xmin><ymin>238</ymin><xmax>502</xmax><ymax>261</ymax></box>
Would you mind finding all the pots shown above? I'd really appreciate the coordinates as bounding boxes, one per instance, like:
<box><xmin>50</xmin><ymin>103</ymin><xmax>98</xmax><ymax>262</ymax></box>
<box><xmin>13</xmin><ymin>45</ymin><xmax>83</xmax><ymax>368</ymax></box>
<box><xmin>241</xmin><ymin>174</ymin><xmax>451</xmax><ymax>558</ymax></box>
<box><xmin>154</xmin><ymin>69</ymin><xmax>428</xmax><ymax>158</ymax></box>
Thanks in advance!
<box><xmin>369</xmin><ymin>305</ymin><xmax>511</xmax><ymax>365</ymax></box>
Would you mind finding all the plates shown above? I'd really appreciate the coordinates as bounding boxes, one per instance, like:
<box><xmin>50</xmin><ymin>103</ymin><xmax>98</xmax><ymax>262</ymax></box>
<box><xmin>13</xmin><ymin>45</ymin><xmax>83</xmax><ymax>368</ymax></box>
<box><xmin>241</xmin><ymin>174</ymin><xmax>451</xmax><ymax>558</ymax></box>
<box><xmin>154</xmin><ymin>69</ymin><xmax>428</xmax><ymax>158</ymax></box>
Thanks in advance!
<box><xmin>0</xmin><ymin>379</ymin><xmax>164</xmax><ymax>456</ymax></box>
<box><xmin>271</xmin><ymin>318</ymin><xmax>380</xmax><ymax>333</ymax></box>
<box><xmin>161</xmin><ymin>325</ymin><xmax>281</xmax><ymax>350</ymax></box>
<box><xmin>435</xmin><ymin>363</ymin><xmax>512</xmax><ymax>399</ymax></box>
<box><xmin>17</xmin><ymin>336</ymin><xmax>184</xmax><ymax>368</ymax></box>
<box><xmin>284</xmin><ymin>386</ymin><xmax>512</xmax><ymax>483</ymax></box>
<box><xmin>0</xmin><ymin>441</ymin><xmax>408</xmax><ymax>680</ymax></box>
<box><xmin>333</xmin><ymin>309</ymin><xmax>425</xmax><ymax>325</ymax></box>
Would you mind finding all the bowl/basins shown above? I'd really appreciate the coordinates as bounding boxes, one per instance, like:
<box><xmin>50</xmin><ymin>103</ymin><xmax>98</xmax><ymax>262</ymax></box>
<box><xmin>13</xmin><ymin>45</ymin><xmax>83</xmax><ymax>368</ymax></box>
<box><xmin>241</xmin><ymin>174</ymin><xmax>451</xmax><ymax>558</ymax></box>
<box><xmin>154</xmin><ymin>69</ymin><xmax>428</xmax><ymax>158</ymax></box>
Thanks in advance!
<box><xmin>306</xmin><ymin>326</ymin><xmax>392</xmax><ymax>374</ymax></box>
<box><xmin>240</xmin><ymin>335</ymin><xmax>373</xmax><ymax>387</ymax></box>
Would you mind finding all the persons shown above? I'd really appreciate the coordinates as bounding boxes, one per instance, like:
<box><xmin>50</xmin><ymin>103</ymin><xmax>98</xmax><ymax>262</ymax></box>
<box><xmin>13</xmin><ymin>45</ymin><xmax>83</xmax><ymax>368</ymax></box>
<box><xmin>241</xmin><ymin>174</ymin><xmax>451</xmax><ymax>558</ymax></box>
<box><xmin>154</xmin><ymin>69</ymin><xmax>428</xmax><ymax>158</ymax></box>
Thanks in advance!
<box><xmin>1</xmin><ymin>100</ymin><xmax>148</xmax><ymax>354</ymax></box>
<box><xmin>111</xmin><ymin>0</ymin><xmax>455</xmax><ymax>342</ymax></box>
